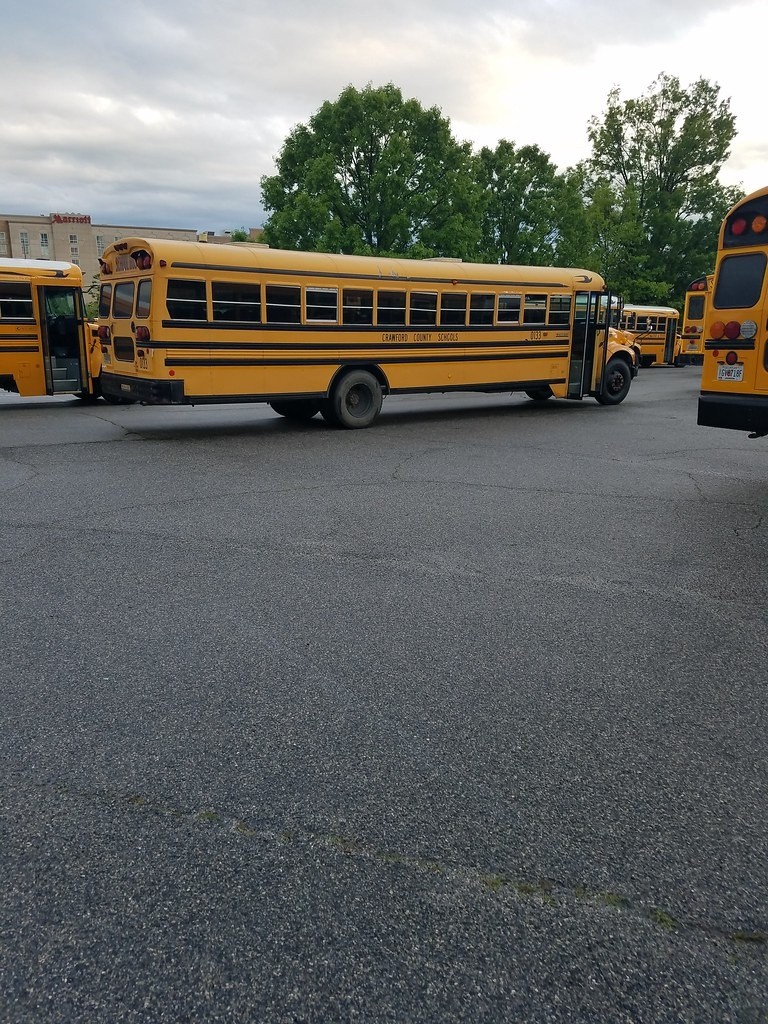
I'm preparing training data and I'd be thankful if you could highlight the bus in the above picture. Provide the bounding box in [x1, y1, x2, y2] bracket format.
[100, 236, 654, 431]
[1, 256, 140, 406]
[696, 184, 768, 439]
[523, 294, 685, 369]
[680, 273, 714, 364]
